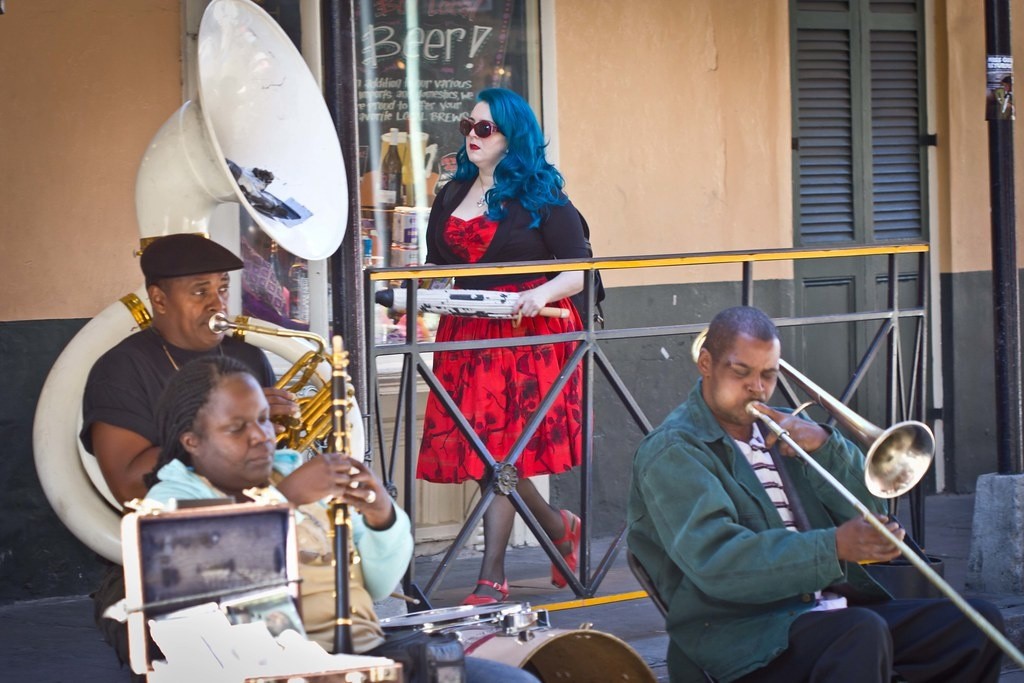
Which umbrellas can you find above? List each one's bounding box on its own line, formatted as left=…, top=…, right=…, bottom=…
left=375, top=288, right=572, bottom=321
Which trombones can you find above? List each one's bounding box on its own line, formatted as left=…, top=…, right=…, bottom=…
left=690, top=326, right=1023, bottom=671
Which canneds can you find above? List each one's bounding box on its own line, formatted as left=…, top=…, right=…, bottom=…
left=390, top=205, right=432, bottom=270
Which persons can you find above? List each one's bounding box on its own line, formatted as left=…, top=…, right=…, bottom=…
left=628, top=307, right=1004, bottom=683
left=378, top=89, right=589, bottom=609
left=75, top=237, right=544, bottom=683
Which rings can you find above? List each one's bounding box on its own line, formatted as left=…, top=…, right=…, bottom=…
left=366, top=490, right=378, bottom=503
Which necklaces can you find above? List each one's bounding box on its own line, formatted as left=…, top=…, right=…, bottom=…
left=155, top=327, right=225, bottom=370
left=477, top=181, right=490, bottom=209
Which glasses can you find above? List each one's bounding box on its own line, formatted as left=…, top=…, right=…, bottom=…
left=459, top=116, right=500, bottom=139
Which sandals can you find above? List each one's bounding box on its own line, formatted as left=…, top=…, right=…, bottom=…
left=464, top=575, right=508, bottom=605
left=550, top=509, right=581, bottom=589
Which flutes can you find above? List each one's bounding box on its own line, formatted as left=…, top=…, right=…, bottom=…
left=331, top=333, right=354, bottom=654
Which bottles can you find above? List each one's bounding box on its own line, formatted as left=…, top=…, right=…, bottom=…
left=362, top=219, right=372, bottom=270
left=287, top=255, right=311, bottom=326
left=382, top=126, right=404, bottom=208
left=269, top=240, right=284, bottom=287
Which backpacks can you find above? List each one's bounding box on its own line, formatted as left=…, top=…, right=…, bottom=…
left=539, top=194, right=606, bottom=313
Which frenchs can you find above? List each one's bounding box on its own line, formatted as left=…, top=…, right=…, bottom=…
left=29, top=0, right=349, bottom=564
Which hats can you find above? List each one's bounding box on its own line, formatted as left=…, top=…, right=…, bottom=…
left=141, top=234, right=243, bottom=279
